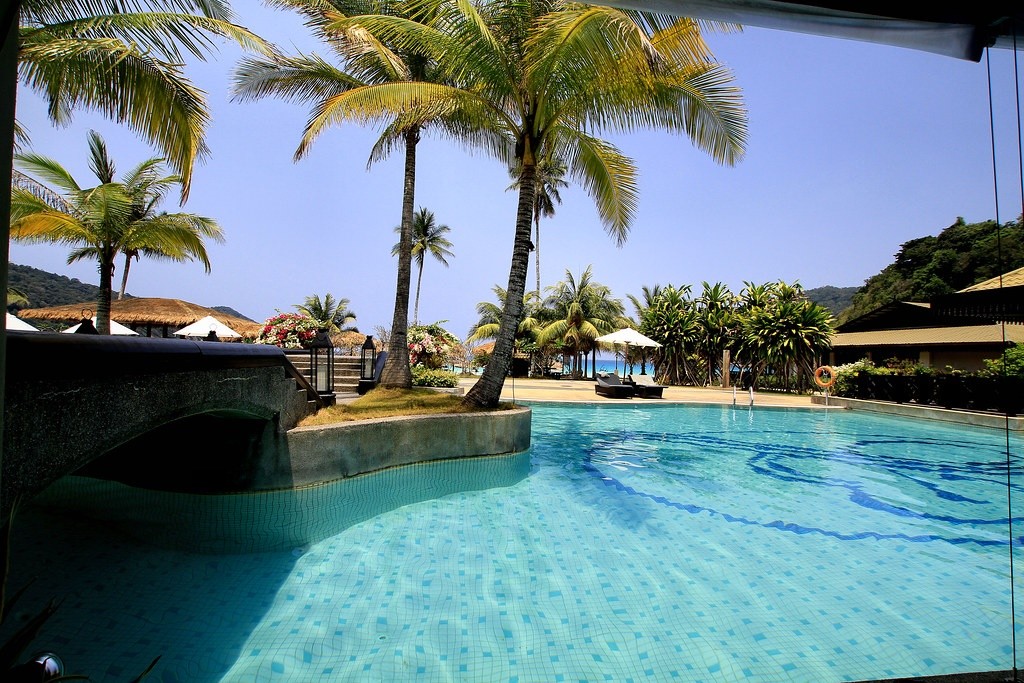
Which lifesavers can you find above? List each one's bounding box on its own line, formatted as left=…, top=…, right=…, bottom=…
left=814, top=366, right=835, bottom=387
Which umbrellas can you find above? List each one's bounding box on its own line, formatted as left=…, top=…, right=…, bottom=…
left=595, top=328, right=663, bottom=381
left=332, top=330, right=376, bottom=355
left=473, top=340, right=531, bottom=359
left=6, top=313, right=39, bottom=331
left=62, top=317, right=139, bottom=335
left=173, top=316, right=243, bottom=338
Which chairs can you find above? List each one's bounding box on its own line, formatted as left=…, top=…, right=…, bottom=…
left=595, top=373, right=634, bottom=399
left=628, top=375, right=669, bottom=399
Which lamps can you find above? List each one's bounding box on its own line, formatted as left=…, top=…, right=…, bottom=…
left=309, top=329, right=334, bottom=394
left=360, top=335, right=376, bottom=380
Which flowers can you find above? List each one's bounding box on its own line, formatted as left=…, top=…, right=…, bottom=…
left=252, top=309, right=317, bottom=350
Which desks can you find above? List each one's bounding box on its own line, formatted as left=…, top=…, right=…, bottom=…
left=622, top=381, right=636, bottom=396
